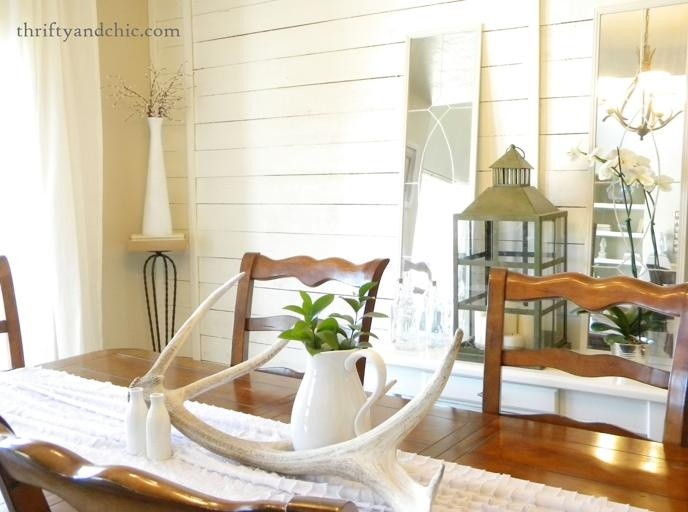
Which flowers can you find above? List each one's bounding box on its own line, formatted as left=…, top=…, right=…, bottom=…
left=593, top=145, right=677, bottom=345
left=568, top=144, right=654, bottom=347
left=101, top=56, right=195, bottom=126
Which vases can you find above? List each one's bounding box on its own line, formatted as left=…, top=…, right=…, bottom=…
left=611, top=337, right=655, bottom=365
left=143, top=113, right=171, bottom=238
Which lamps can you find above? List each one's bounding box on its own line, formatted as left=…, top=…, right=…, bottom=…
left=602, top=9, right=685, bottom=139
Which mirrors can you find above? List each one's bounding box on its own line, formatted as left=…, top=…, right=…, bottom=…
left=576, top=1, right=686, bottom=358
left=396, top=23, right=485, bottom=345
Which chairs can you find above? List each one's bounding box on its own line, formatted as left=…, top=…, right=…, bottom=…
left=475, top=264, right=687, bottom=448
left=231, top=251, right=391, bottom=379
left=0, top=417, right=352, bottom=512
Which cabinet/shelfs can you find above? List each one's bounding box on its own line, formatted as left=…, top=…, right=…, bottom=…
left=565, top=149, right=681, bottom=346
left=453, top=144, right=568, bottom=364
left=396, top=368, right=666, bottom=444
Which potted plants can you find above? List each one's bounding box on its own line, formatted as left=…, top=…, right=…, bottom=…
left=281, top=280, right=393, bottom=451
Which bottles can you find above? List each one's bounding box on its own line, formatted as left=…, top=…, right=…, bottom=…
left=123, top=385, right=172, bottom=460
